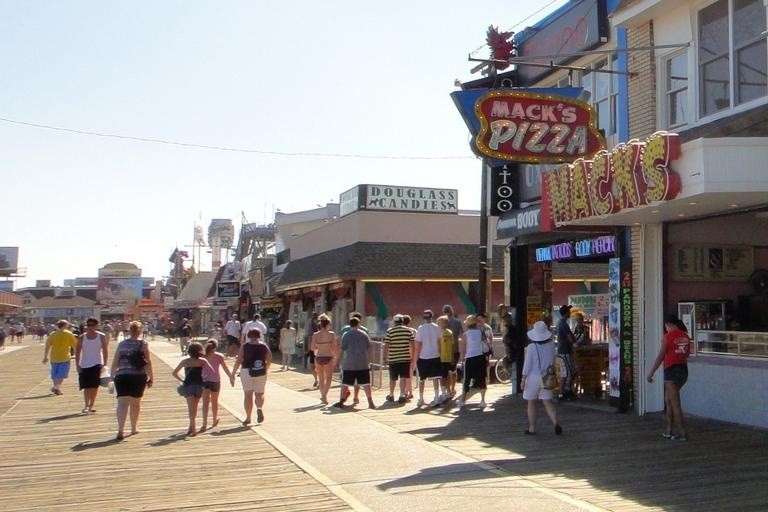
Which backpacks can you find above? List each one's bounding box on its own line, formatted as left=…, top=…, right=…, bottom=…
left=244, top=342, right=265, bottom=377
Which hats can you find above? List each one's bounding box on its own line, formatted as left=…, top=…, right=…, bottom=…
left=560, top=304, right=572, bottom=315
left=527, top=321, right=553, bottom=342
left=393, top=310, right=481, bottom=326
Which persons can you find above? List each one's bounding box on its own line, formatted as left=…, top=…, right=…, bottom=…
left=200, top=339, right=234, bottom=431
left=0, top=321, right=59, bottom=350
left=75, top=317, right=109, bottom=412
left=230, top=328, right=272, bottom=424
left=304, top=312, right=377, bottom=409
left=109, top=320, right=154, bottom=440
left=556, top=304, right=576, bottom=399
left=647, top=313, right=691, bottom=442
left=41, top=320, right=77, bottom=395
left=382, top=303, right=521, bottom=409
left=172, top=342, right=216, bottom=436
left=572, top=312, right=592, bottom=346
left=520, top=320, right=563, bottom=436
left=65, top=309, right=296, bottom=370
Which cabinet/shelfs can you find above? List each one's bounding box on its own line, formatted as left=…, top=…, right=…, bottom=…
left=678, top=300, right=728, bottom=357
left=697, top=330, right=768, bottom=358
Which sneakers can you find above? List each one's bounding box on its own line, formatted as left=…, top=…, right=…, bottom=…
left=257, top=408, right=264, bottom=424
left=524, top=429, right=535, bottom=434
left=661, top=430, right=673, bottom=439
left=554, top=424, right=563, bottom=434
left=333, top=390, right=487, bottom=411
left=671, top=433, right=688, bottom=441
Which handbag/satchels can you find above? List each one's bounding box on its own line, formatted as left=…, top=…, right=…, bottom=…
left=128, top=351, right=147, bottom=369
left=177, top=383, right=185, bottom=396
left=541, top=373, right=560, bottom=390
